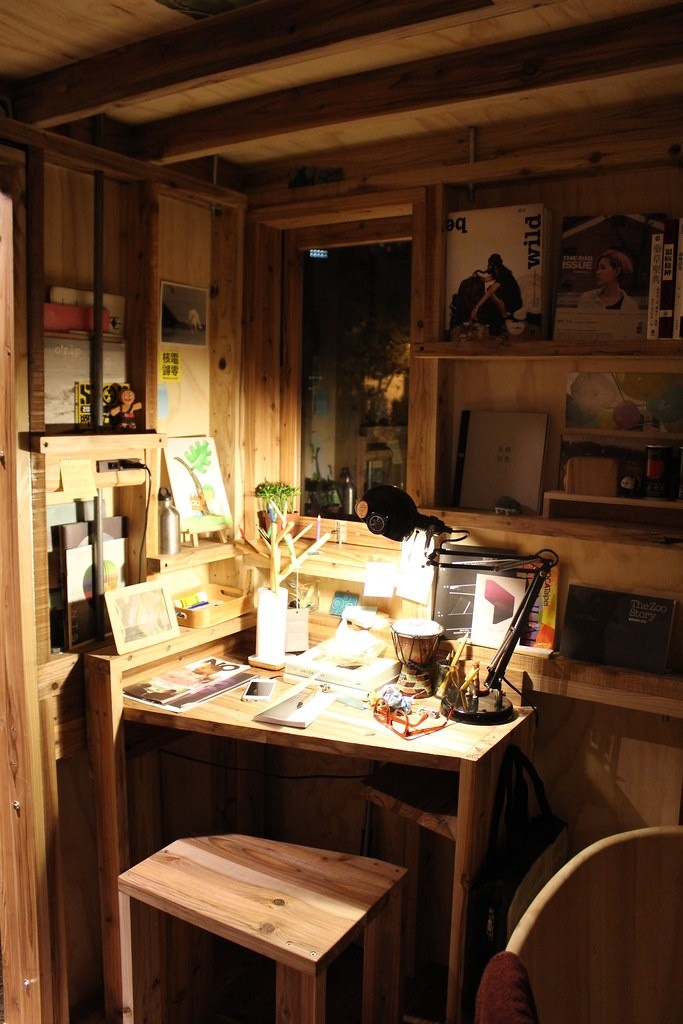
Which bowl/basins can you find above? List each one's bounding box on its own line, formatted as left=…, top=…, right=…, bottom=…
left=506, top=322, right=525, bottom=335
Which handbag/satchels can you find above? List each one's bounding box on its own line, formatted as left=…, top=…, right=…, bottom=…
left=468, top=745, right=570, bottom=957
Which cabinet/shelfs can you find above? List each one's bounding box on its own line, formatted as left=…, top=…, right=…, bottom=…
left=0, top=114, right=250, bottom=664
left=410, top=156, right=683, bottom=719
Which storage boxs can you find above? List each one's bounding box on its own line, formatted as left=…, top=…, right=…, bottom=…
left=173, top=583, right=252, bottom=629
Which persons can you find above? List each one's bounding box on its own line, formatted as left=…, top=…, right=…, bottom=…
left=471, top=254, right=523, bottom=321
left=578, top=250, right=639, bottom=311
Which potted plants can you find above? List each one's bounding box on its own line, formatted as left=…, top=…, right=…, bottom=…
left=304, top=475, right=342, bottom=519
left=254, top=482, right=302, bottom=541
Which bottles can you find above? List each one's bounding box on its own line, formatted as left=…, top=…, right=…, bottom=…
left=158, top=488, right=182, bottom=555
left=339, top=467, right=355, bottom=516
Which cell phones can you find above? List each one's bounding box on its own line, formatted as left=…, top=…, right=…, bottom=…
left=242, top=679, right=275, bottom=702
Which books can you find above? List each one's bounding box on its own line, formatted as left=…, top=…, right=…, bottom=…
left=254, top=672, right=338, bottom=728
left=451, top=410, right=550, bottom=515
left=553, top=213, right=683, bottom=340
left=433, top=544, right=559, bottom=659
left=559, top=584, right=677, bottom=676
left=60, top=515, right=129, bottom=653
left=443, top=204, right=554, bottom=342
left=122, top=656, right=258, bottom=713
left=283, top=646, right=401, bottom=710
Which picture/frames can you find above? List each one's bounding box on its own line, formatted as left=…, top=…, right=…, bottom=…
left=104, top=579, right=181, bottom=655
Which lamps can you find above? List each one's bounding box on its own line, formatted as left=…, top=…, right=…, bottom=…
left=353, top=483, right=561, bottom=726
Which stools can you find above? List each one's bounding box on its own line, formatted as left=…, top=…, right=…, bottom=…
left=117, top=833, right=411, bottom=1024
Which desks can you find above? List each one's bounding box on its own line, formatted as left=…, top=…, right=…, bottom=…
left=87, top=609, right=537, bottom=1024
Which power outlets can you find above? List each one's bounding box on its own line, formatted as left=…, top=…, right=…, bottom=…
left=118, top=457, right=139, bottom=471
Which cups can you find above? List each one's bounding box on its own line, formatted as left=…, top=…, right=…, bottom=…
left=642, top=443, right=675, bottom=501
